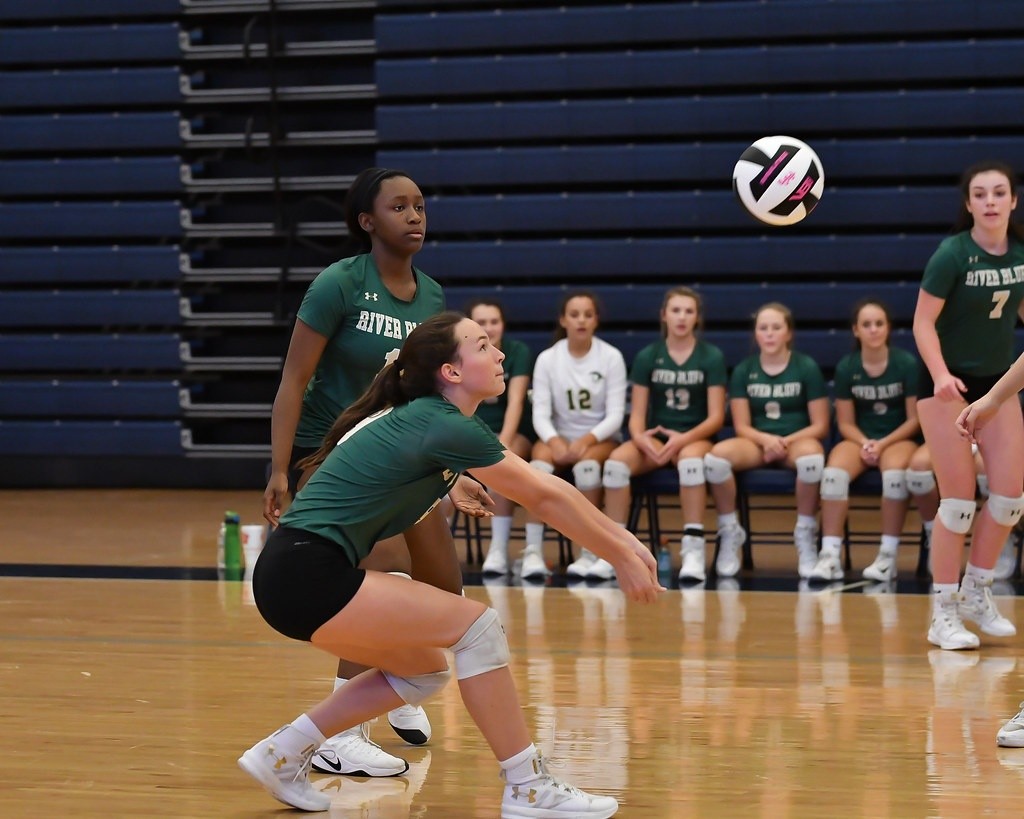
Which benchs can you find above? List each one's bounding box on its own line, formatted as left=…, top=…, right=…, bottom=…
left=0, top=0, right=1024, bottom=456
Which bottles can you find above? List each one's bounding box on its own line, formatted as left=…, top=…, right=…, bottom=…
left=215, top=521, right=226, bottom=568
left=656, top=537, right=673, bottom=590
left=224, top=510, right=241, bottom=569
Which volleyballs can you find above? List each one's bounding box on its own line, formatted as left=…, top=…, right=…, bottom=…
left=731, top=134, right=826, bottom=227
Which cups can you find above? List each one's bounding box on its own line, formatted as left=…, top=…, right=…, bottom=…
left=241, top=525, right=264, bottom=568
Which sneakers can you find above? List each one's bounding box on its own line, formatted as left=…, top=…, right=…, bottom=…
left=237, top=724, right=330, bottom=811
left=679, top=535, right=705, bottom=581
left=311, top=778, right=408, bottom=811
left=928, top=650, right=979, bottom=684
left=811, top=545, right=845, bottom=582
left=715, top=522, right=746, bottom=577
left=501, top=749, right=619, bottom=819
left=997, top=701, right=1024, bottom=747
left=310, top=720, right=408, bottom=777
left=482, top=543, right=507, bottom=574
left=585, top=557, right=616, bottom=579
left=717, top=578, right=746, bottom=621
left=520, top=545, right=545, bottom=578
left=925, top=537, right=934, bottom=574
left=794, top=525, right=820, bottom=578
left=483, top=576, right=508, bottom=595
left=800, top=581, right=841, bottom=602
left=863, top=582, right=896, bottom=601
left=927, top=594, right=980, bottom=650
left=566, top=551, right=597, bottom=578
left=523, top=578, right=545, bottom=600
left=388, top=703, right=431, bottom=744
left=567, top=579, right=624, bottom=601
left=979, top=657, right=1016, bottom=679
left=993, top=534, right=1018, bottom=580
left=679, top=580, right=706, bottom=608
left=863, top=548, right=897, bottom=581
left=399, top=750, right=431, bottom=794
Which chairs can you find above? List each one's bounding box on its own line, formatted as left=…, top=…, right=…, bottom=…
left=449, top=418, right=1024, bottom=587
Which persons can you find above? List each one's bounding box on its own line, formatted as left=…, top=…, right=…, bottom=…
left=236, top=309, right=668, bottom=819
left=589, top=286, right=730, bottom=580
left=262, top=167, right=494, bottom=778
left=704, top=303, right=830, bottom=578
left=811, top=300, right=926, bottom=581
left=518, top=294, right=625, bottom=579
left=466, top=297, right=536, bottom=574
left=907, top=439, right=1017, bottom=579
left=909, top=168, right=1024, bottom=649
